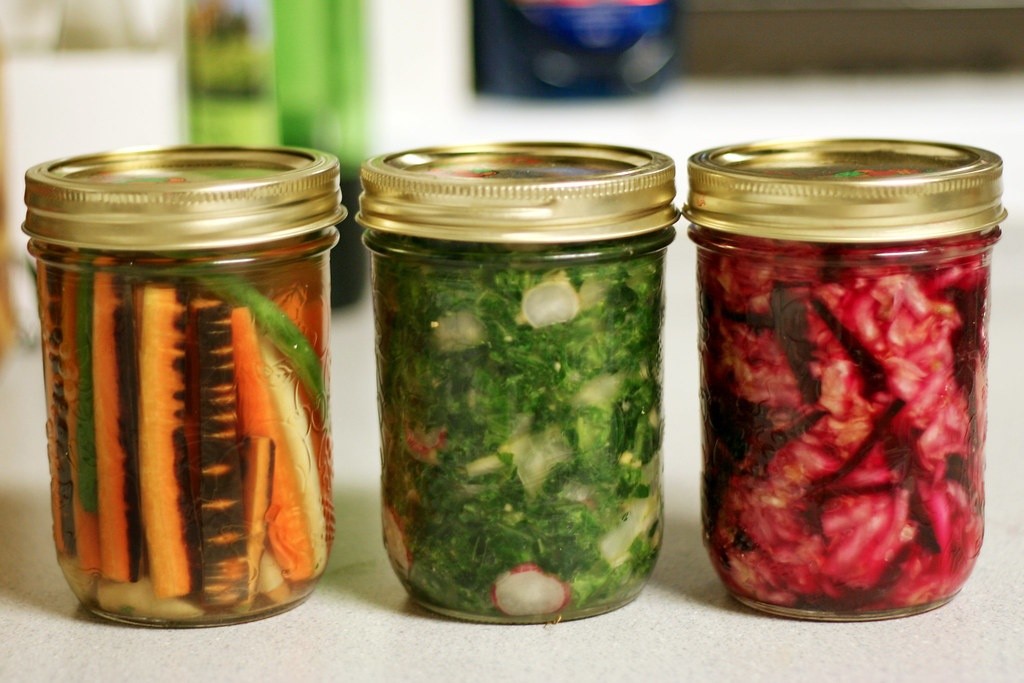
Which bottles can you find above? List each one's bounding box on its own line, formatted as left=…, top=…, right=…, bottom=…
left=682, top=137, right=1009, bottom=622
left=183, top=0, right=370, bottom=308
left=19, top=142, right=348, bottom=629
left=352, top=140, right=682, bottom=624
